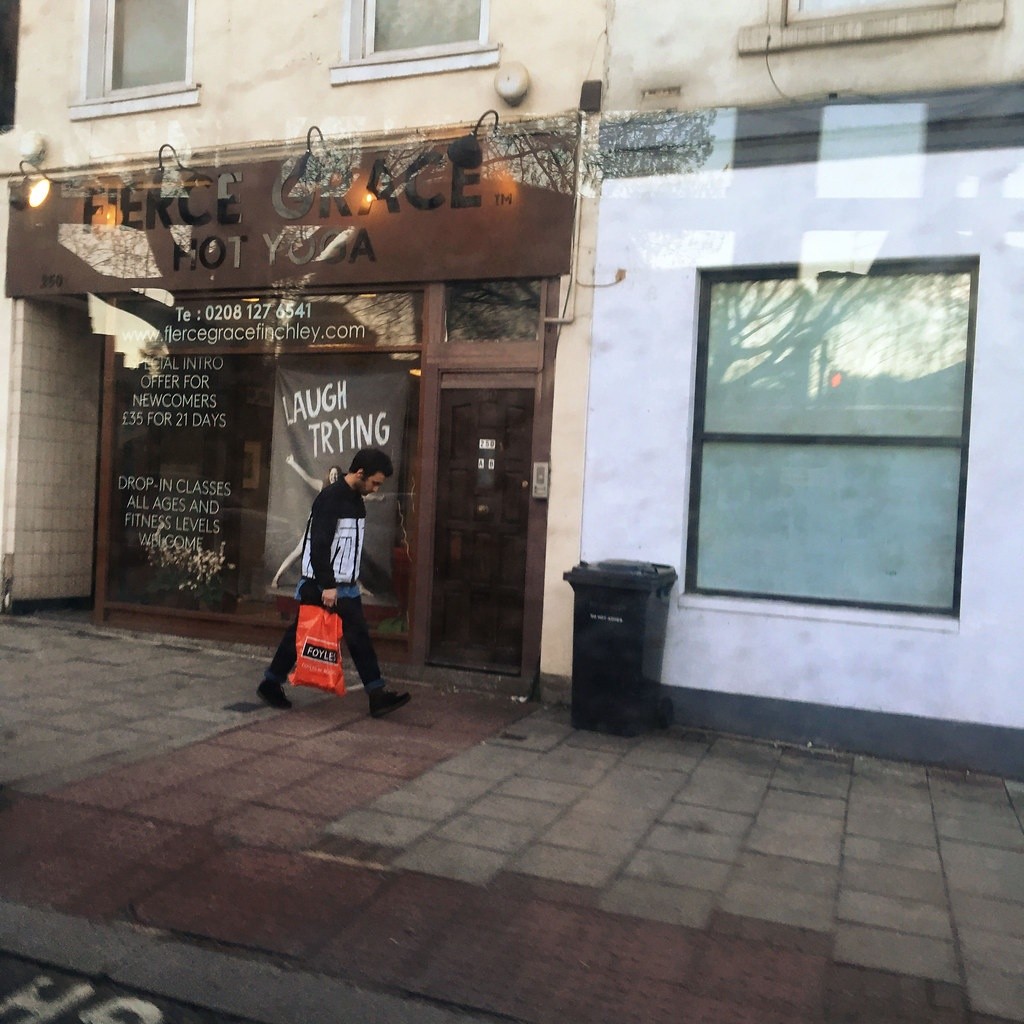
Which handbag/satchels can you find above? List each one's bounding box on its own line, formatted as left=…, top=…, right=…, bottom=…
left=287, top=604, right=347, bottom=696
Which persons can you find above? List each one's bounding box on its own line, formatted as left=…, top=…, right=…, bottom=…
left=270, top=454, right=385, bottom=597
left=256, top=448, right=411, bottom=719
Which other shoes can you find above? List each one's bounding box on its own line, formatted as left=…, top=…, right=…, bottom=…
left=256, top=680, right=292, bottom=709
left=369, top=688, right=411, bottom=718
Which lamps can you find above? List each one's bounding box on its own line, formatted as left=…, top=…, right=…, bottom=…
left=447, top=110, right=499, bottom=168
left=296, top=125, right=334, bottom=182
left=19, top=160, right=72, bottom=209
left=149, top=143, right=195, bottom=200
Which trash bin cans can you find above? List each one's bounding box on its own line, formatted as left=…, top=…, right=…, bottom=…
left=563, top=558, right=680, bottom=735
